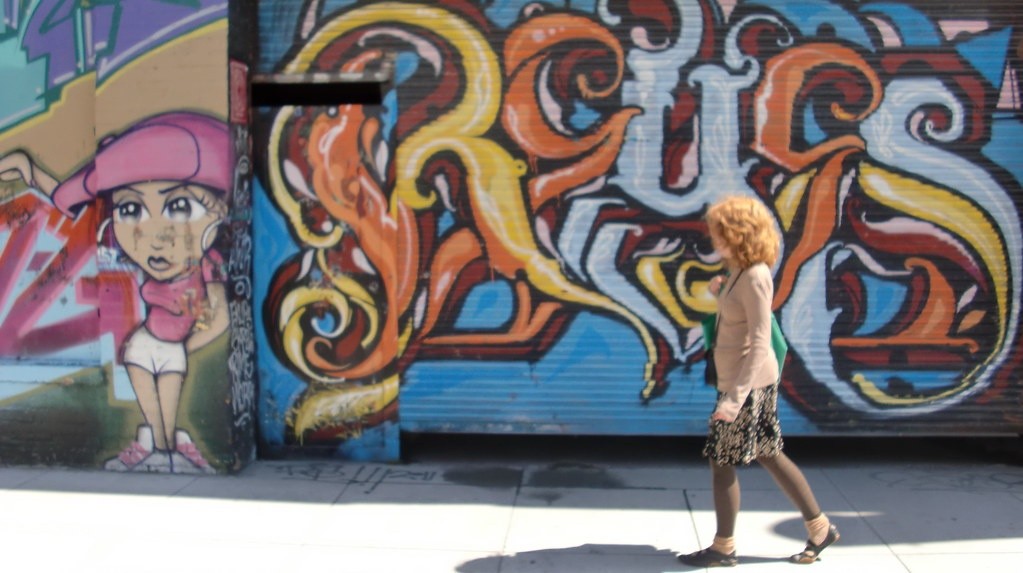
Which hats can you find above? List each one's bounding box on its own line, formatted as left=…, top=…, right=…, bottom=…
left=51, top=111, right=241, bottom=219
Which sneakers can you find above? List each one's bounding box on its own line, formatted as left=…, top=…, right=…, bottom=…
left=170, top=431, right=215, bottom=474
left=678, top=547, right=738, bottom=567
left=102, top=426, right=170, bottom=473
left=790, top=526, right=840, bottom=564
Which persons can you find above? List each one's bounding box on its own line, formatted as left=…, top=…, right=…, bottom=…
left=678, top=194, right=841, bottom=568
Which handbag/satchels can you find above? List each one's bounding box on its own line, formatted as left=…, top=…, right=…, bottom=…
left=700, top=313, right=789, bottom=387
